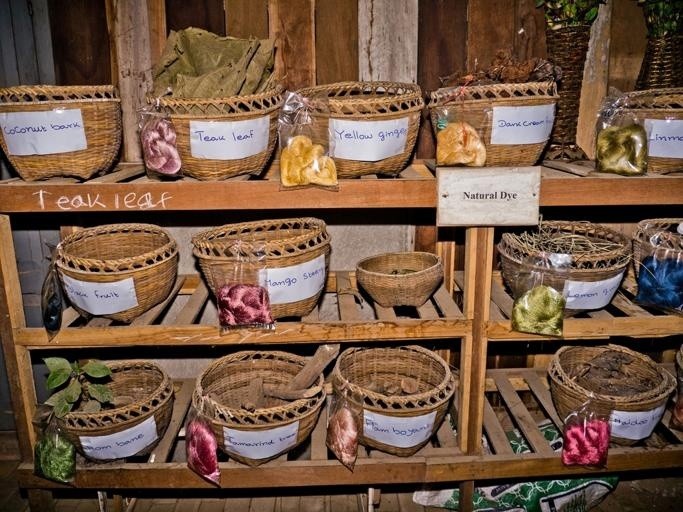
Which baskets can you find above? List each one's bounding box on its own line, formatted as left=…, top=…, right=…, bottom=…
left=53, top=223, right=180, bottom=325
left=497, top=219, right=631, bottom=319
left=545, top=342, right=677, bottom=447
left=190, top=215, right=331, bottom=321
left=50, top=359, right=176, bottom=464
left=143, top=84, right=285, bottom=183
left=428, top=78, right=560, bottom=169
left=190, top=350, right=328, bottom=468
left=289, top=81, right=425, bottom=176
left=331, top=342, right=456, bottom=458
left=354, top=250, right=445, bottom=308
left=0, top=83, right=122, bottom=183
left=631, top=216, right=682, bottom=313
left=612, top=88, right=683, bottom=177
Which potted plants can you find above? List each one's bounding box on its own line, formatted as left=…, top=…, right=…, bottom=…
left=533, top=0, right=682, bottom=162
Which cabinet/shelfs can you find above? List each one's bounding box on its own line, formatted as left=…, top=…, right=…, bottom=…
left=1, top=155, right=682, bottom=511
